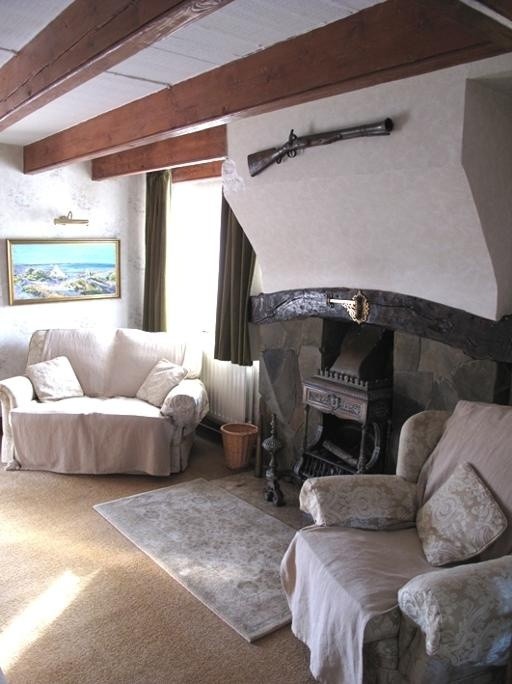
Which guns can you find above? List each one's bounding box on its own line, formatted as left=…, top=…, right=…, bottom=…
left=247, top=117, right=394, bottom=177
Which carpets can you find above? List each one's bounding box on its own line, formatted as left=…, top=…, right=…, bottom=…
left=94, top=474, right=300, bottom=643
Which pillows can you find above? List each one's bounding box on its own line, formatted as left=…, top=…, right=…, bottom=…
left=26, top=355, right=84, bottom=403
left=415, top=460, right=508, bottom=567
left=135, top=355, right=189, bottom=408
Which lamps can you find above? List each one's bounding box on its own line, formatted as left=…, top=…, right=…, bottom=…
left=54, top=212, right=89, bottom=226
left=199, top=351, right=255, bottom=428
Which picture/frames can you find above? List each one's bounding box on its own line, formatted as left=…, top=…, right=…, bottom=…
left=5, top=237, right=121, bottom=305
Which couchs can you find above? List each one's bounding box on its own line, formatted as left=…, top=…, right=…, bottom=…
left=0, top=327, right=209, bottom=477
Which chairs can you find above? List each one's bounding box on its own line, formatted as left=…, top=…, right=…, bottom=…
left=278, top=398, right=511, bottom=684
